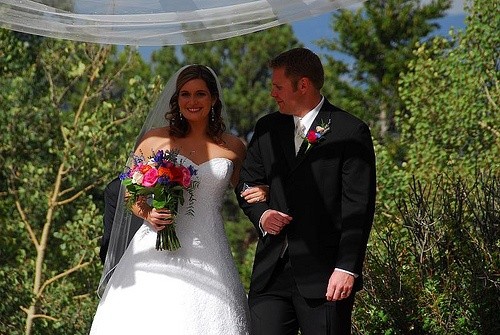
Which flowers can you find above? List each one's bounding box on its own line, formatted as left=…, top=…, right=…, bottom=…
left=299, top=118, right=332, bottom=155
left=120, top=144, right=202, bottom=252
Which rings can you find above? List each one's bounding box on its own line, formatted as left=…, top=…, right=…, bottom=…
left=256, top=198, right=259, bottom=202
left=341, top=291, right=347, bottom=295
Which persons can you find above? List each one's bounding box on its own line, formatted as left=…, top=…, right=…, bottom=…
left=234, top=48, right=378, bottom=334
left=89, top=63, right=270, bottom=334
